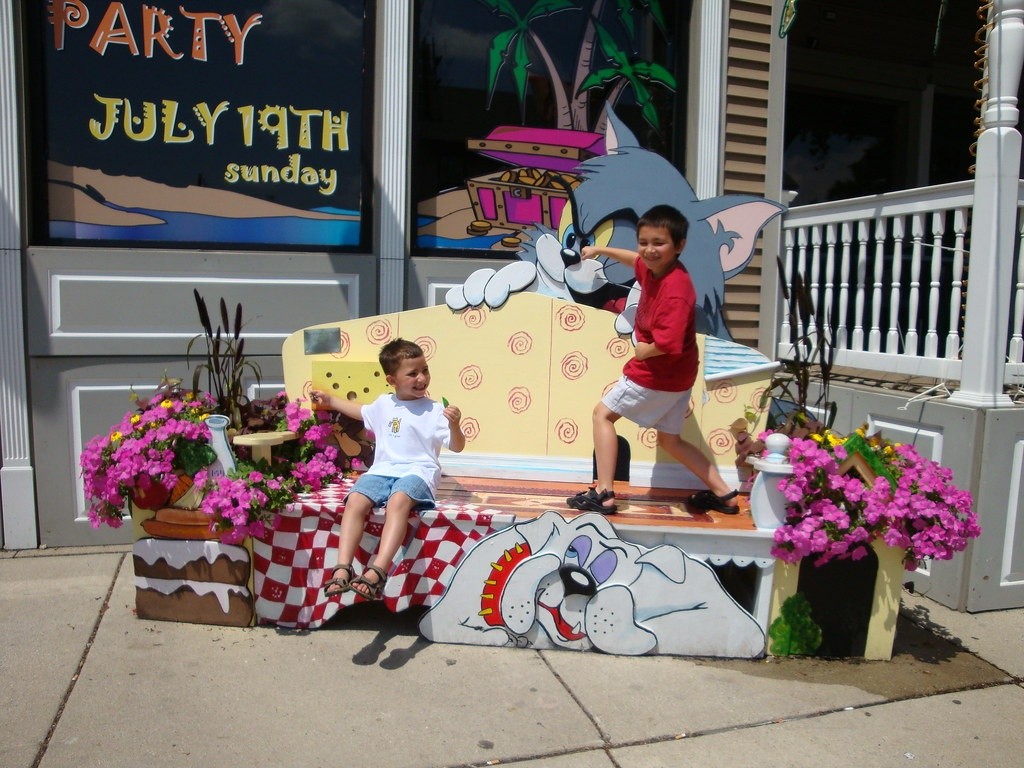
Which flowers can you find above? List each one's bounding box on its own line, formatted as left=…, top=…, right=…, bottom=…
left=79, top=285, right=377, bottom=547
left=755, top=254, right=984, bottom=572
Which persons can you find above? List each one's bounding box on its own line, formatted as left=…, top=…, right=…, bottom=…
left=309, top=339, right=465, bottom=600
left=566, top=205, right=740, bottom=515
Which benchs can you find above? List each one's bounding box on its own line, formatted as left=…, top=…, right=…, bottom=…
left=232, top=293, right=797, bottom=661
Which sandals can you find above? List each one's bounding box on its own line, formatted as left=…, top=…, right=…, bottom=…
left=689, top=488, right=740, bottom=514
left=349, top=566, right=387, bottom=600
left=566, top=486, right=617, bottom=515
left=324, top=565, right=355, bottom=596
